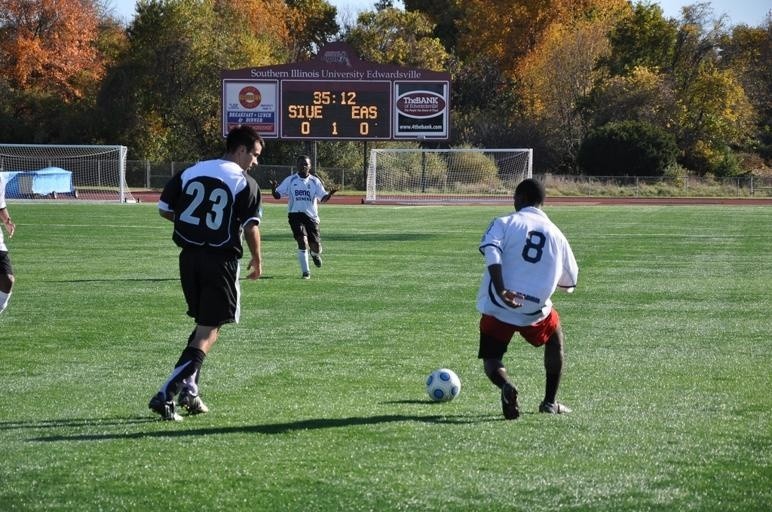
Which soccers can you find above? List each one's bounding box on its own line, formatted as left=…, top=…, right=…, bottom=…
left=427, top=367, right=461, bottom=405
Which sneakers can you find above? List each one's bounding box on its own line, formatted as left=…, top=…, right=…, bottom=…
left=149, top=392, right=186, bottom=423
left=302, top=272, right=311, bottom=280
left=538, top=399, right=573, bottom=415
left=180, top=386, right=209, bottom=414
left=502, top=382, right=520, bottom=420
left=309, top=249, right=323, bottom=268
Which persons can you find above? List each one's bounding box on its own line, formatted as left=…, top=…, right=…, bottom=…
left=478, top=178, right=579, bottom=419
left=269, top=155, right=339, bottom=280
left=0, top=175, right=16, bottom=314
left=149, top=124, right=263, bottom=422
